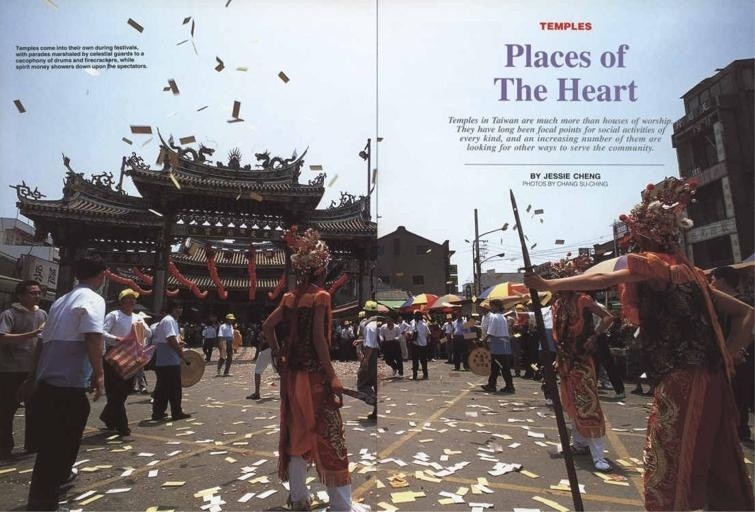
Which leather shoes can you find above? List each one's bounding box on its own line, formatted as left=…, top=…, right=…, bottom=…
left=120, top=428, right=131, bottom=434
left=100, top=414, right=114, bottom=430
left=152, top=413, right=168, bottom=420
left=172, top=413, right=190, bottom=419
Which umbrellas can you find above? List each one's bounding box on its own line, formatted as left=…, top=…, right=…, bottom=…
left=396, top=278, right=550, bottom=314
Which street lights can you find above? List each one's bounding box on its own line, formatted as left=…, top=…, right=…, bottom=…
left=117, top=155, right=132, bottom=191
left=359, top=136, right=377, bottom=300
left=471, top=222, right=510, bottom=298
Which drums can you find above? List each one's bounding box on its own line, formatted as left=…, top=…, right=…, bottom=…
left=464, top=340, right=491, bottom=375
left=180, top=348, right=205, bottom=388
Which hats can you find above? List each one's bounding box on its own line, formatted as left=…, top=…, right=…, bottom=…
left=118, top=288, right=140, bottom=302
left=478, top=303, right=491, bottom=311
left=225, top=313, right=237, bottom=320
left=618, top=172, right=700, bottom=249
left=358, top=311, right=366, bottom=318
left=279, top=225, right=331, bottom=276
left=362, top=300, right=378, bottom=312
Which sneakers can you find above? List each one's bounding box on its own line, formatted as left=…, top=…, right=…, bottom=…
left=567, top=446, right=584, bottom=454
left=631, top=388, right=642, bottom=394
left=500, top=386, right=515, bottom=393
left=616, top=387, right=625, bottom=399
left=644, top=392, right=652, bottom=396
left=246, top=393, right=260, bottom=399
left=481, top=385, right=496, bottom=391
left=593, top=457, right=612, bottom=472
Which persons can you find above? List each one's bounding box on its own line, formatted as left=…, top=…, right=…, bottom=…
left=352, top=300, right=378, bottom=428
left=707, top=264, right=755, bottom=441
left=150, top=297, right=192, bottom=421
left=261, top=246, right=372, bottom=512
left=243, top=302, right=287, bottom=400
left=87, top=310, right=244, bottom=394
left=522, top=216, right=755, bottom=511
left=0, top=278, right=49, bottom=458
left=544, top=271, right=616, bottom=471
left=335, top=300, right=658, bottom=395
left=98, top=287, right=153, bottom=437
left=14, top=252, right=109, bottom=512
